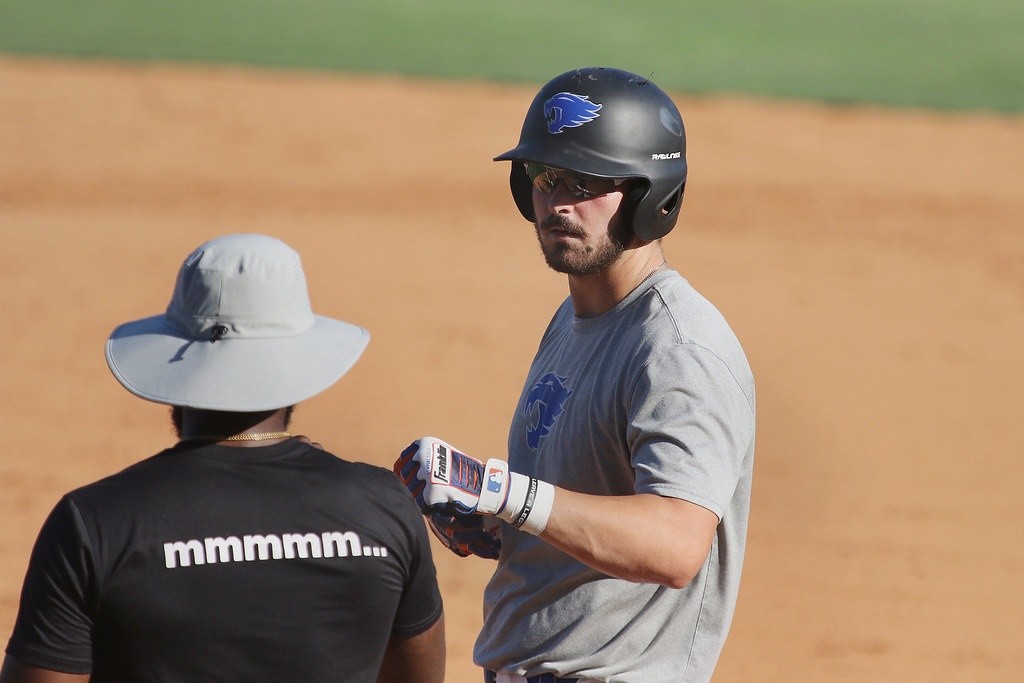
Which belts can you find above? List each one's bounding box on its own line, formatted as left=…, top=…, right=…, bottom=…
left=484, top=668, right=579, bottom=683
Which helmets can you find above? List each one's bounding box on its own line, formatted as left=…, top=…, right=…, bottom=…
left=492, top=67, right=689, bottom=241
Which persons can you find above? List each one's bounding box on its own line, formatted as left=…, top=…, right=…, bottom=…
left=0, top=233, right=446, bottom=683
left=392, top=67, right=757, bottom=683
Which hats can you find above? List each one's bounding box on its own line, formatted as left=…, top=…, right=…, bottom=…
left=105, top=234, right=371, bottom=411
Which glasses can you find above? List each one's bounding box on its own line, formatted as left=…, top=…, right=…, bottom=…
left=523, top=161, right=634, bottom=198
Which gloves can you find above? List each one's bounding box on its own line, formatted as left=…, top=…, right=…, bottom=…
left=393, top=436, right=555, bottom=558
